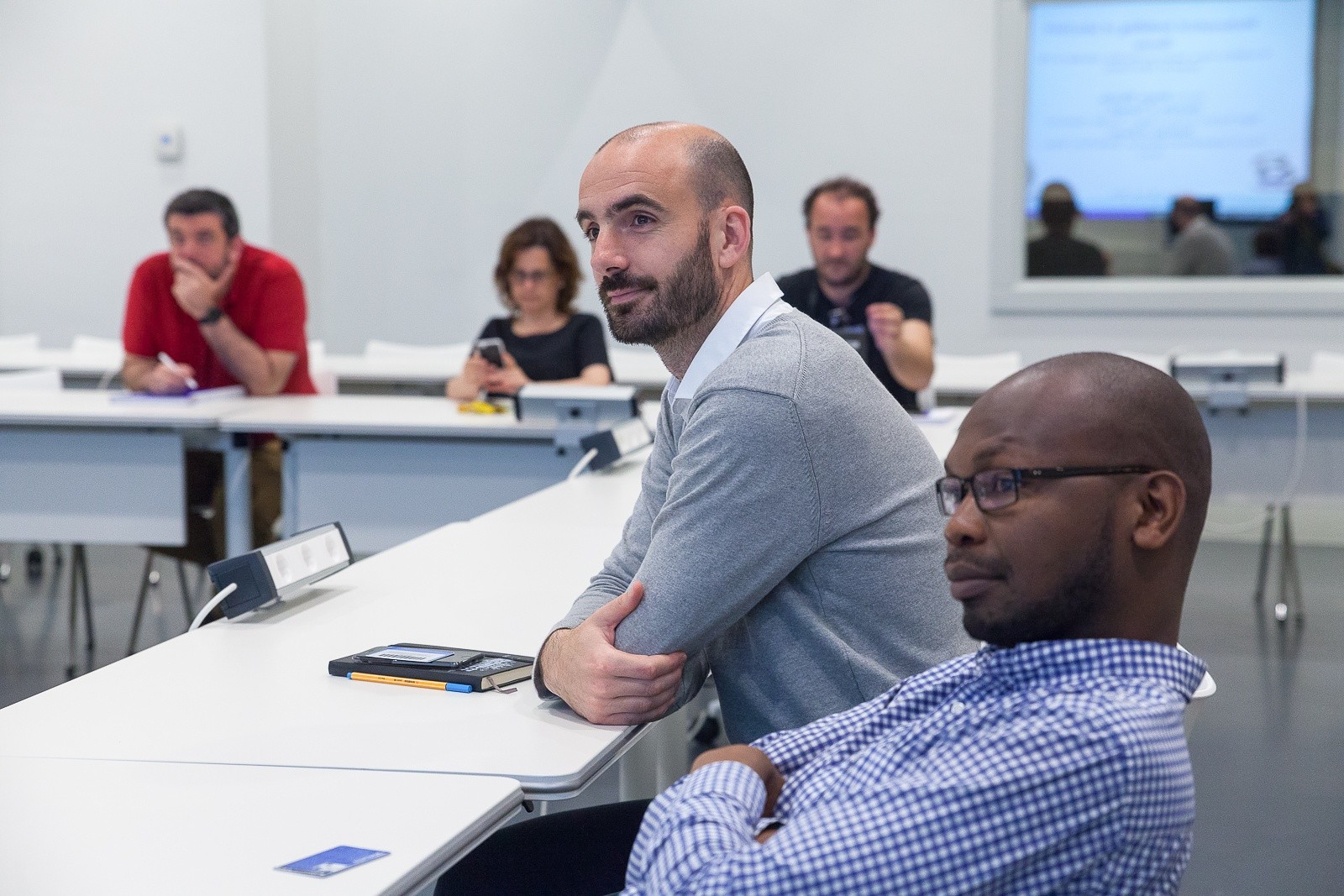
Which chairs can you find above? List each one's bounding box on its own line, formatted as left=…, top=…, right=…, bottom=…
left=935, top=355, right=1021, bottom=394
left=1169, top=351, right=1343, bottom=637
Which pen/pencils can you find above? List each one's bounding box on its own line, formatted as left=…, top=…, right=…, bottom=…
left=158, top=350, right=202, bottom=391
left=347, top=672, right=472, bottom=693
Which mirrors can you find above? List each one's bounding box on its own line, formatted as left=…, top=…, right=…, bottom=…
left=993, top=0, right=1344, bottom=323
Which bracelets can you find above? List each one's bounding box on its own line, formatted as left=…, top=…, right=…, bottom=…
left=196, top=308, right=222, bottom=325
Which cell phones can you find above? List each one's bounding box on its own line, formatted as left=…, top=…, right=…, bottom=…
left=476, top=339, right=506, bottom=369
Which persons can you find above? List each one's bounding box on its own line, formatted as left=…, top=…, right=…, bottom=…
left=613, top=354, right=1210, bottom=896
left=777, top=179, right=933, bottom=412
left=444, top=217, right=612, bottom=402
left=1256, top=182, right=1344, bottom=275
left=1160, top=199, right=1250, bottom=278
left=122, top=190, right=318, bottom=565
left=1027, top=183, right=1105, bottom=276
left=430, top=121, right=983, bottom=896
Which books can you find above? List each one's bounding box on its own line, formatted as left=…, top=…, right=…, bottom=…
left=327, top=643, right=535, bottom=692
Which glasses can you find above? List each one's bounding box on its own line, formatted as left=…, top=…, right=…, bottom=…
left=934, top=465, right=1159, bottom=518
left=509, top=269, right=555, bottom=284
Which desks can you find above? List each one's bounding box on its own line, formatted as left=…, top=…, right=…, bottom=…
left=1, top=758, right=524, bottom=894
left=220, top=393, right=661, bottom=557
left=1, top=391, right=243, bottom=427
left=0, top=522, right=660, bottom=817
left=473, top=427, right=972, bottom=522
left=637, top=399, right=972, bottom=439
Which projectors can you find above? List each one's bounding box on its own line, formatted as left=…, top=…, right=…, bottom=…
left=1169, top=351, right=1282, bottom=383
